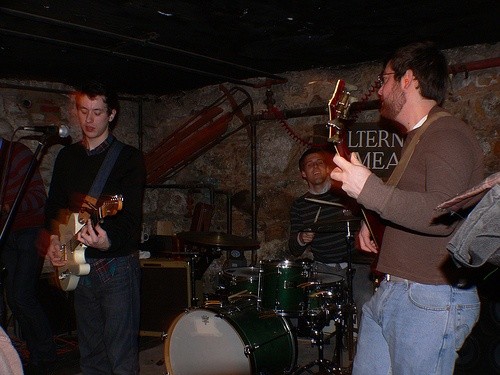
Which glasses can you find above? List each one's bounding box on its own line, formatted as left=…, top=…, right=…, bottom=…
left=377, top=72, right=395, bottom=85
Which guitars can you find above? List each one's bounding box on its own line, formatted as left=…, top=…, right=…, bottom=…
left=326, top=80, right=380, bottom=249
left=54, top=193, right=123, bottom=291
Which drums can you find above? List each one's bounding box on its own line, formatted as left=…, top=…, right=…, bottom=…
left=259, top=258, right=309, bottom=318
left=160, top=297, right=299, bottom=375
left=223, top=267, right=260, bottom=294
left=308, top=273, right=349, bottom=309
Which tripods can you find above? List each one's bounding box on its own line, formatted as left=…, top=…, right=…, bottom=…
left=293, top=322, right=344, bottom=375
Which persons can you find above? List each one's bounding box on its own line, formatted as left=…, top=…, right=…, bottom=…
left=288, top=148, right=372, bottom=332
left=0, top=136, right=57, bottom=375
left=329, top=41, right=483, bottom=375
left=46, top=79, right=146, bottom=375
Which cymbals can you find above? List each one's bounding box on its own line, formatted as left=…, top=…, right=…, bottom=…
left=174, top=230, right=259, bottom=248
left=302, top=211, right=363, bottom=226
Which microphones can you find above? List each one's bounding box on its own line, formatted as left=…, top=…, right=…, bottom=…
left=18, top=125, right=70, bottom=138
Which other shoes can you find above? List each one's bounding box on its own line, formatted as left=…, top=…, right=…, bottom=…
left=344, top=313, right=358, bottom=344
left=311, top=320, right=337, bottom=343
